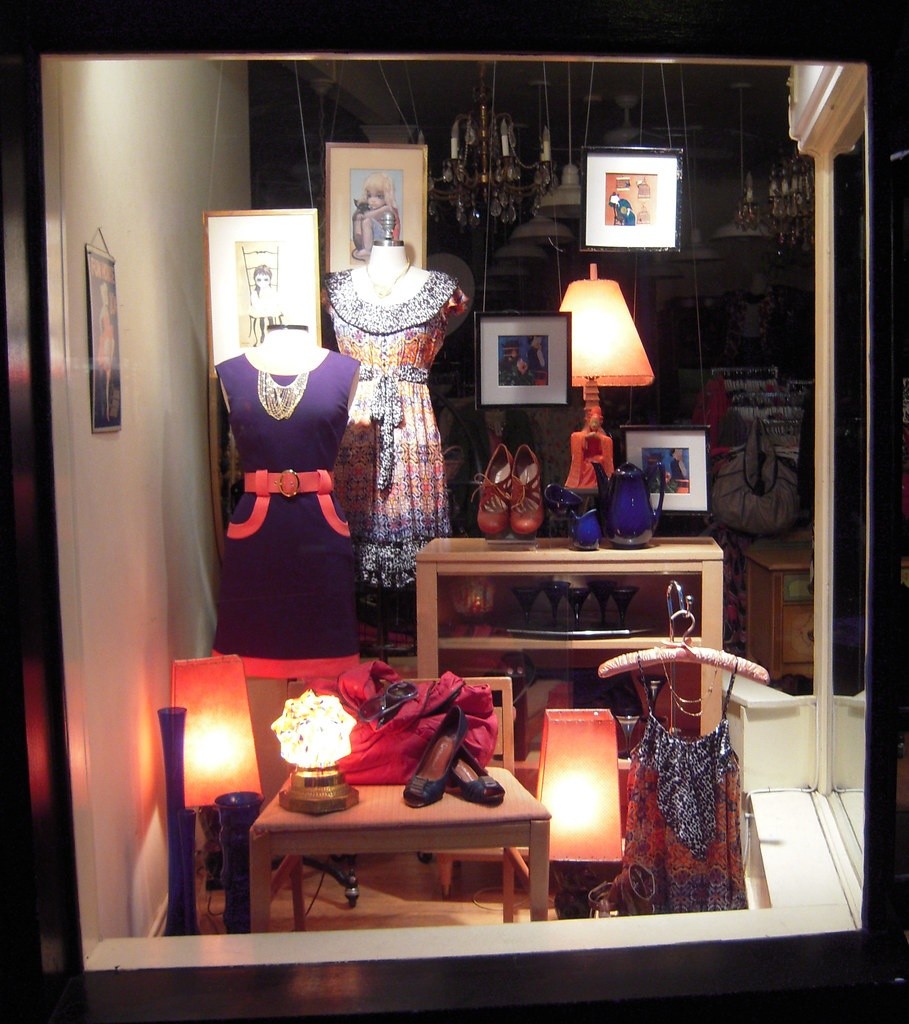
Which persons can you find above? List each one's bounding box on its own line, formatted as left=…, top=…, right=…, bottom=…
left=564, top=407, right=614, bottom=489
left=211, top=325, right=359, bottom=679
left=320, top=246, right=469, bottom=586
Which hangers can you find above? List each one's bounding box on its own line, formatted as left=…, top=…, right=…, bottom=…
left=597, top=612, right=772, bottom=687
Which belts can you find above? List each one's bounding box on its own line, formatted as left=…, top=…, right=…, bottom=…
left=225, top=469, right=350, bottom=541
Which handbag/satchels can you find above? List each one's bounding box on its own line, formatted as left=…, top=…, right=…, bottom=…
left=334, top=657, right=499, bottom=784
left=712, top=417, right=801, bottom=537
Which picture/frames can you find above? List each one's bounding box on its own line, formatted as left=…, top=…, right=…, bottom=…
left=473, top=311, right=573, bottom=406
left=204, top=205, right=322, bottom=376
left=325, top=142, right=430, bottom=274
left=580, top=145, right=684, bottom=252
left=622, top=424, right=713, bottom=515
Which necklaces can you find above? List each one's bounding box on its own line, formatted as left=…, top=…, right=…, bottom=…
left=659, top=648, right=719, bottom=716
left=256, top=349, right=312, bottom=425
left=365, top=256, right=410, bottom=299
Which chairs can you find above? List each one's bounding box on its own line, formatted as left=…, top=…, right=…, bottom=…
left=397, top=677, right=531, bottom=893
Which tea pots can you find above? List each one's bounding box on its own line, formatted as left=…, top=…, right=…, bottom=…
left=591, top=461, right=666, bottom=545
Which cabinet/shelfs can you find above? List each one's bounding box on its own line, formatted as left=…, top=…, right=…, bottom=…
left=745, top=545, right=815, bottom=680
left=414, top=537, right=725, bottom=735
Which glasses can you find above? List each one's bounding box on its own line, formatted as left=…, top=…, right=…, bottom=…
left=358, top=679, right=419, bottom=730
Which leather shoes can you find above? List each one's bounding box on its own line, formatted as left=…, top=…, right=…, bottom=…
left=471, top=443, right=545, bottom=537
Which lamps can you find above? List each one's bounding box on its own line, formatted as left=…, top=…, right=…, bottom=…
left=557, top=263, right=654, bottom=494
left=492, top=243, right=553, bottom=268
left=512, top=213, right=574, bottom=248
left=426, top=60, right=559, bottom=223
left=710, top=76, right=769, bottom=242
left=536, top=62, right=578, bottom=218
left=271, top=690, right=359, bottom=813
left=488, top=265, right=528, bottom=278
left=535, top=710, right=624, bottom=919
left=676, top=121, right=723, bottom=264
left=170, top=656, right=262, bottom=890
left=738, top=147, right=815, bottom=251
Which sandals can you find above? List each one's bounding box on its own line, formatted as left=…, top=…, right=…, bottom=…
left=404, top=703, right=506, bottom=808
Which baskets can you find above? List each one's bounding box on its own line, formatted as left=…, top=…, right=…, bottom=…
left=444, top=446, right=464, bottom=481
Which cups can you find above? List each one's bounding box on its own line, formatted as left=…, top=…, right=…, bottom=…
left=570, top=509, right=602, bottom=550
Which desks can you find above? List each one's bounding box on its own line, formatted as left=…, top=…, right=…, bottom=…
left=247, top=766, right=551, bottom=935
